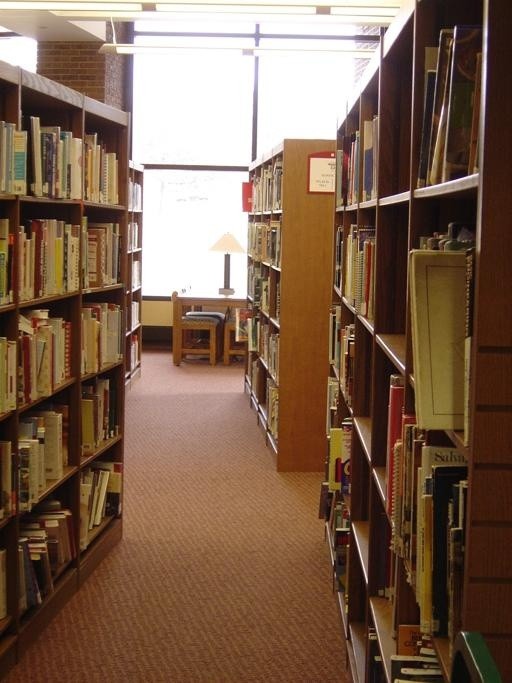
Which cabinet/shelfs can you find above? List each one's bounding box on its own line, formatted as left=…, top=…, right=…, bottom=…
left=0, top=64, right=132, bottom=680
left=0, top=61, right=143, bottom=679
left=129, top=158, right=143, bottom=390
left=246, top=139, right=337, bottom=474
left=320, top=2, right=510, bottom=683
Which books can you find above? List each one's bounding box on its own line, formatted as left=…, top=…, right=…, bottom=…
left=234, top=162, right=282, bottom=440
left=317, top=24, right=483, bottom=683
left=0, top=108, right=141, bottom=631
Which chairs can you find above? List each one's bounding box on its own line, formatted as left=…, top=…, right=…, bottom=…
left=168, top=283, right=251, bottom=361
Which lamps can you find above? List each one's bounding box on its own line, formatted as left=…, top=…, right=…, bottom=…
left=211, top=228, right=241, bottom=294
